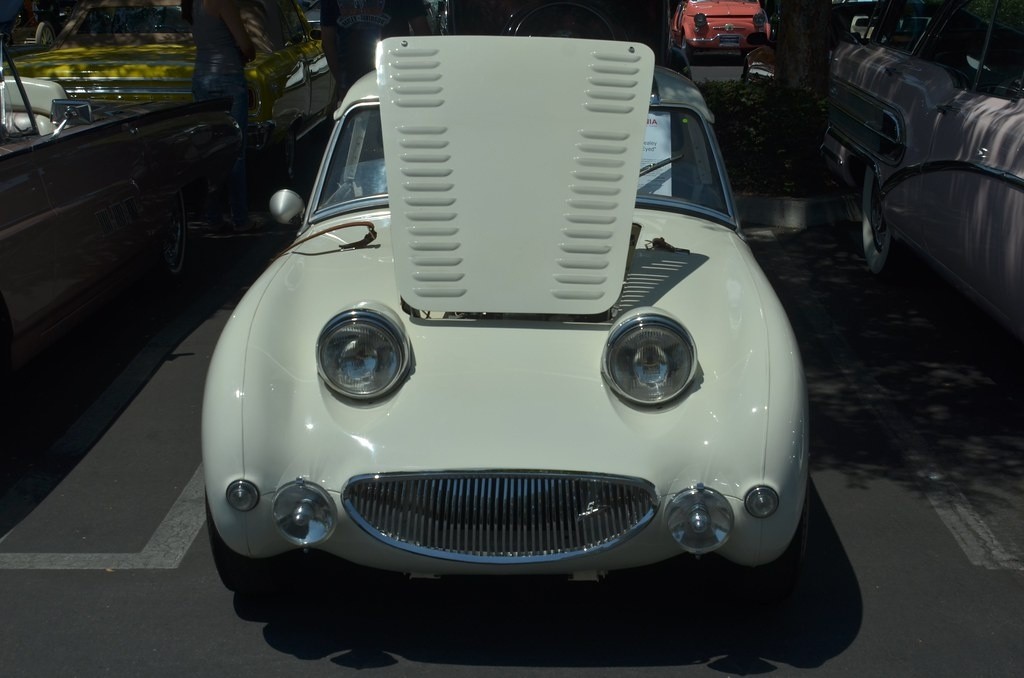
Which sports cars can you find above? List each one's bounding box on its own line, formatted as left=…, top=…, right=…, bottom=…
left=200, top=33, right=814, bottom=616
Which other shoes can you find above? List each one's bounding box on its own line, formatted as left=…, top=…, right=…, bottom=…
left=231, top=214, right=266, bottom=233
left=201, top=221, right=233, bottom=238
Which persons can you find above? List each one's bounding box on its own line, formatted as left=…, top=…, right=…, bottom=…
left=320, top=0, right=432, bottom=108
left=191, top=0, right=256, bottom=159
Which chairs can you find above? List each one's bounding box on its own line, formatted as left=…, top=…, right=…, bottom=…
left=4, top=75, right=70, bottom=136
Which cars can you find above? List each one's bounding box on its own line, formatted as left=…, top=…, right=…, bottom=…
left=0, top=40, right=245, bottom=368
left=1, top=0, right=349, bottom=159
left=664, top=0, right=778, bottom=66
left=811, top=0, right=1023, bottom=351
